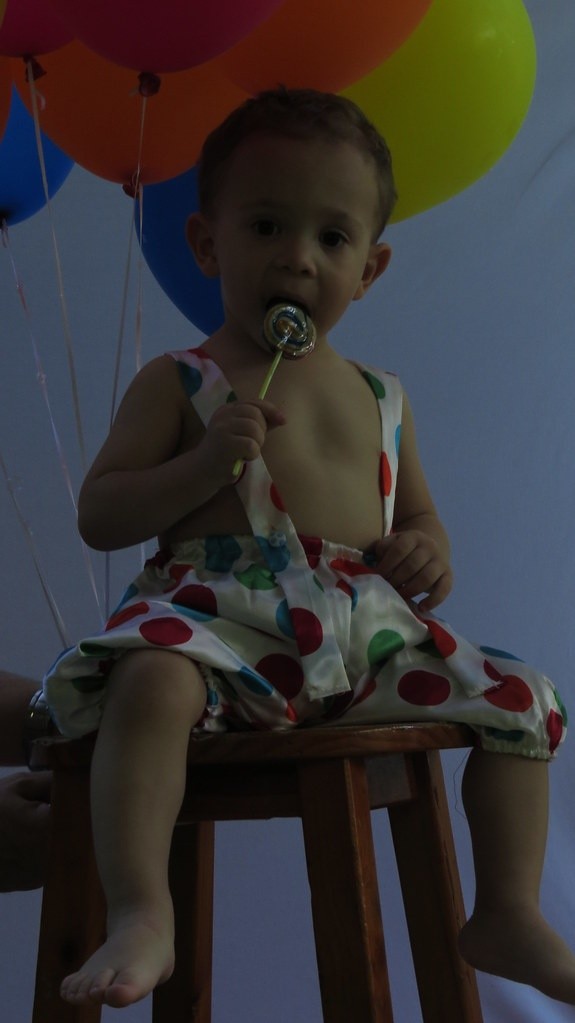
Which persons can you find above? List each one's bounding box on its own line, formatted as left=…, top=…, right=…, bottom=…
left=59, top=88, right=575, bottom=1011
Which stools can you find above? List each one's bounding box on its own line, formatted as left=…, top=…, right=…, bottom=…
left=33, top=721, right=482, bottom=1022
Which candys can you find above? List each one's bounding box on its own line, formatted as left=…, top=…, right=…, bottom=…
left=225, top=305, right=317, bottom=474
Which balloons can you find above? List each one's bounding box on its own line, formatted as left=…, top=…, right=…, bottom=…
left=0, top=1, right=539, bottom=343
